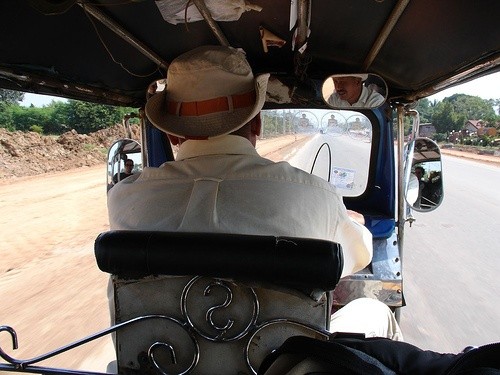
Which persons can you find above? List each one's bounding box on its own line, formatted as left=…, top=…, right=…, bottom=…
left=326, top=74, right=385, bottom=107
left=107, top=44, right=405, bottom=343
left=414, top=167, right=432, bottom=204
left=111, top=159, right=134, bottom=185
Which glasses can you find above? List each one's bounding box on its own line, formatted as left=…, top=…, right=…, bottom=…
left=126, top=164, right=134, bottom=167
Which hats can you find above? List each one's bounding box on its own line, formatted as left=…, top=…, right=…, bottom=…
left=144, top=44, right=270, bottom=140
left=331, top=74, right=368, bottom=81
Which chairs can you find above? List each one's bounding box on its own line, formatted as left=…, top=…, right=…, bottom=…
left=94, top=230, right=344, bottom=375
